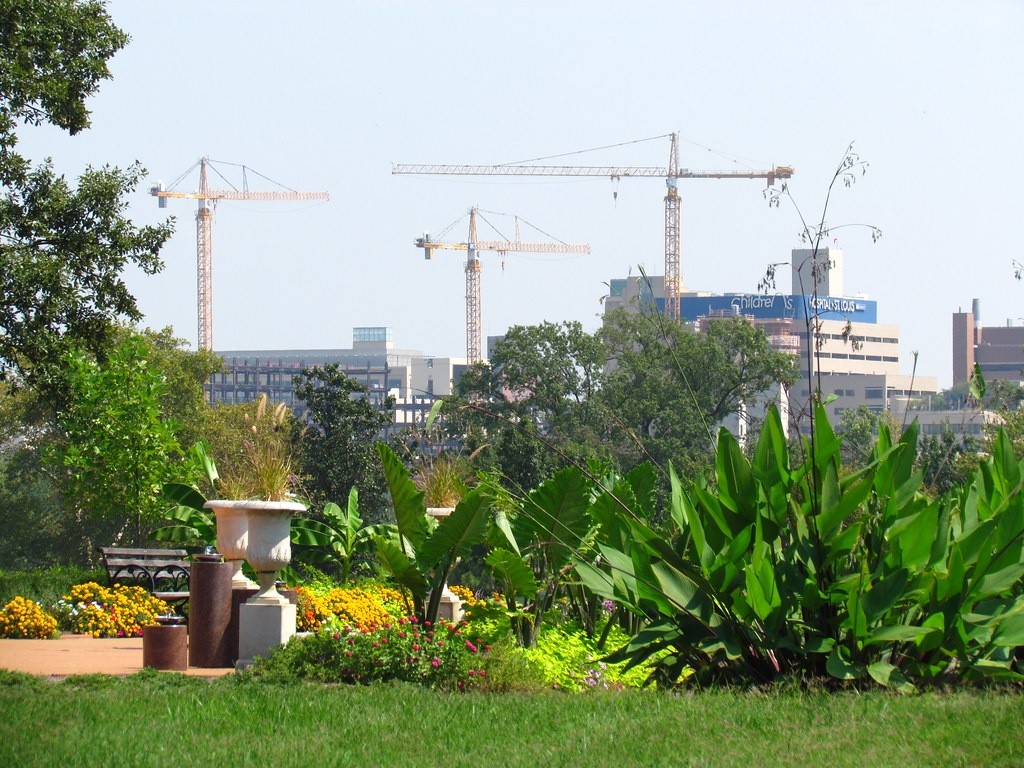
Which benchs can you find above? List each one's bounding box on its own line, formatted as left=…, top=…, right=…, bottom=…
left=97, top=546, right=191, bottom=632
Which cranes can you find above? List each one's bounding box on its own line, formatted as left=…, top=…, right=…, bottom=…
left=412, top=207, right=590, bottom=366
left=149, top=156, right=331, bottom=350
left=390, top=131, right=794, bottom=322
